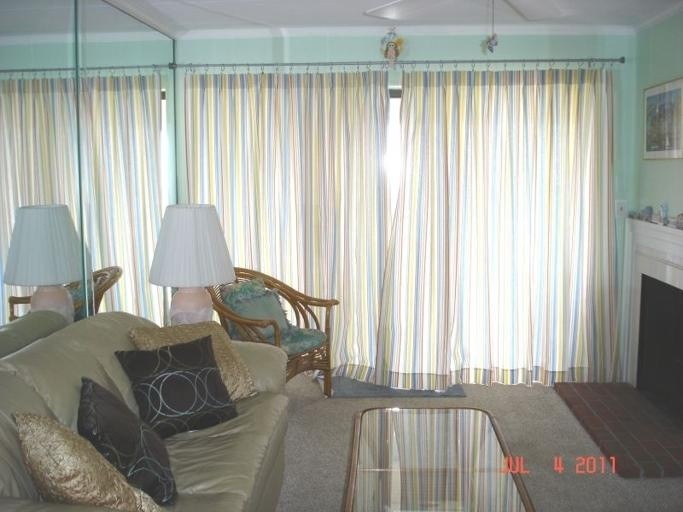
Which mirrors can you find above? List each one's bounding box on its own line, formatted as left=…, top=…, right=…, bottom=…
left=0, top=0, right=177, bottom=329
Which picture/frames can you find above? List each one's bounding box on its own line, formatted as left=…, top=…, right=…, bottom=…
left=642, top=78, right=682, bottom=160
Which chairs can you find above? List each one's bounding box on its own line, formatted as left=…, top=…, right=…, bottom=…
left=204, top=268, right=338, bottom=396
left=8, top=265, right=122, bottom=321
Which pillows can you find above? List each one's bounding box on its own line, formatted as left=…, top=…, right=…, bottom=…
left=115, top=334, right=237, bottom=438
left=11, top=411, right=155, bottom=510
left=129, top=320, right=256, bottom=400
left=79, top=377, right=178, bottom=507
left=230, top=290, right=287, bottom=335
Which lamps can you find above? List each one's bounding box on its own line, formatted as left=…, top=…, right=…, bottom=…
left=2, top=205, right=93, bottom=319
left=149, top=204, right=237, bottom=326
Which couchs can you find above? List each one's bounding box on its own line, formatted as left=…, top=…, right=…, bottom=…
left=0, top=313, right=292, bottom=511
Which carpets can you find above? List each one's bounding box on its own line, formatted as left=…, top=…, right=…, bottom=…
left=333, top=369, right=465, bottom=397
